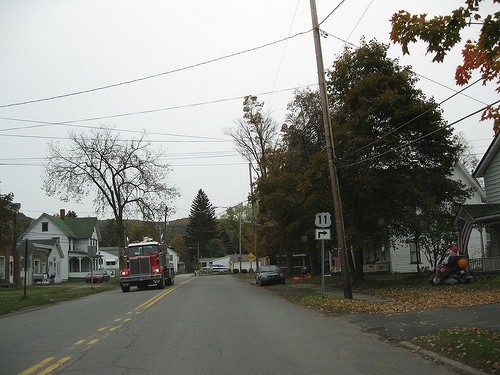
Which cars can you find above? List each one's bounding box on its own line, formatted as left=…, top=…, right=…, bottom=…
left=255, top=265, right=286, bottom=286
left=86, top=270, right=110, bottom=283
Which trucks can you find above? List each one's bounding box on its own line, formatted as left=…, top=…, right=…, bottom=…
left=119, top=233, right=175, bottom=293
